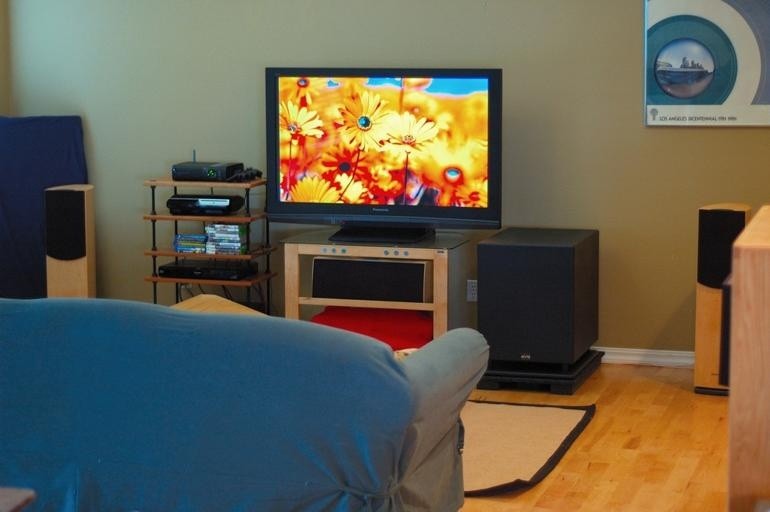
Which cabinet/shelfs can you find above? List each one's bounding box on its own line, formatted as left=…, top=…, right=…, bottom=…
left=143, top=174, right=277, bottom=319
left=280, top=229, right=473, bottom=351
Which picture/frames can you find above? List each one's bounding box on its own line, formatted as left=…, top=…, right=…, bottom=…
left=644, top=1, right=769, bottom=128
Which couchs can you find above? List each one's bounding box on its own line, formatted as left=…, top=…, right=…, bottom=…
left=0, top=296, right=490, bottom=512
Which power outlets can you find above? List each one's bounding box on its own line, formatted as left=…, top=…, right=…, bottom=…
left=466, top=280, right=479, bottom=304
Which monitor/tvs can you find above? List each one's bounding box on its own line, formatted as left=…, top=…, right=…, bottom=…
left=265, top=67, right=503, bottom=244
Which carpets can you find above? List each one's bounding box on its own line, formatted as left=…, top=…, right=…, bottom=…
left=457, top=398, right=597, bottom=497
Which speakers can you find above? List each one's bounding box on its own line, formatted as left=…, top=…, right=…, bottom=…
left=44, top=185, right=96, bottom=298
left=694, top=203, right=753, bottom=396
